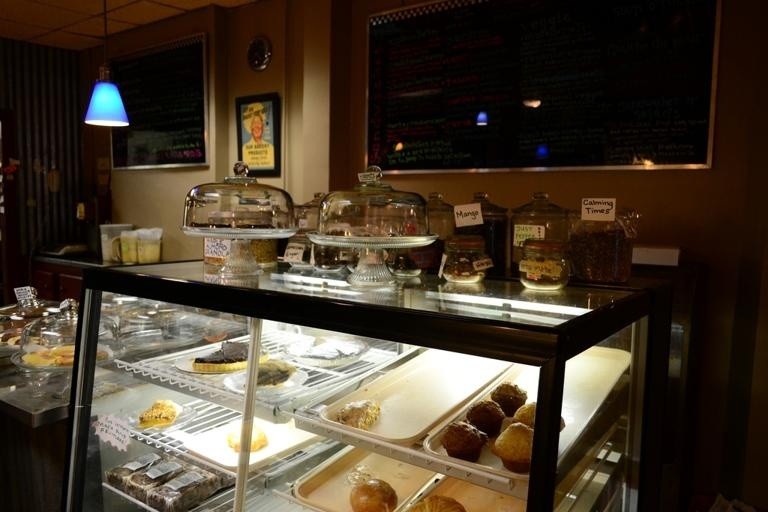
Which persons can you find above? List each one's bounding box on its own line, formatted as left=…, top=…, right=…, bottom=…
left=239, top=104, right=275, bottom=170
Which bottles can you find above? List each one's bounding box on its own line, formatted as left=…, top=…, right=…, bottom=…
left=301, top=191, right=640, bottom=291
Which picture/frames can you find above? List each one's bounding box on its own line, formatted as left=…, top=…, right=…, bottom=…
left=235, top=91, right=281, bottom=178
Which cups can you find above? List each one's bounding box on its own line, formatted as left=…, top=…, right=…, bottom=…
left=136, top=239, right=161, bottom=264
left=100, top=224, right=134, bottom=262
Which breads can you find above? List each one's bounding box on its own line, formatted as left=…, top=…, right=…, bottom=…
left=104, top=450, right=234, bottom=512
left=228, top=427, right=268, bottom=451
left=440, top=381, right=566, bottom=475
left=407, top=495, right=466, bottom=512
left=137, top=401, right=175, bottom=426
left=192, top=341, right=296, bottom=386
left=20, top=346, right=108, bottom=366
left=349, top=479, right=398, bottom=511
left=332, top=399, right=381, bottom=430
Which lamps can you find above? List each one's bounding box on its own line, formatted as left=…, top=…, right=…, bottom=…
left=474, top=109, right=489, bottom=127
left=83, top=0, right=129, bottom=128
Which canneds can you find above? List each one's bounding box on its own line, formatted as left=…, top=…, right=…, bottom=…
left=518, top=238, right=568, bottom=290
left=443, top=233, right=485, bottom=284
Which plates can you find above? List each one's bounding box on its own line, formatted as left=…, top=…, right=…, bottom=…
left=225, top=367, right=307, bottom=395
left=174, top=353, right=236, bottom=375
left=284, top=338, right=367, bottom=366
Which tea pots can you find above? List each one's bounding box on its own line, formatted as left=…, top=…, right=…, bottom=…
left=111, top=229, right=137, bottom=264
